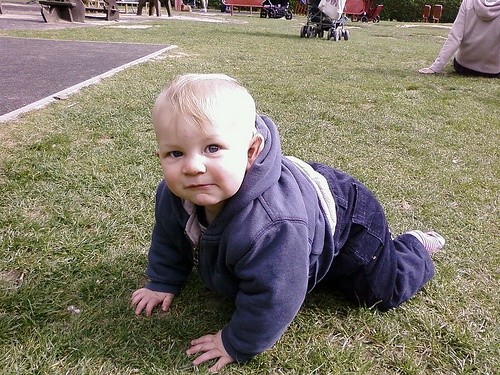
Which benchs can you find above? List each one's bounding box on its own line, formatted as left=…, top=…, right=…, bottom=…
left=38, top=0, right=121, bottom=24
left=222, top=0, right=264, bottom=16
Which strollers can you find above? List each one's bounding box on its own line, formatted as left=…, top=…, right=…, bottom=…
left=298, top=0, right=355, bottom=42
left=260, top=1, right=293, bottom=22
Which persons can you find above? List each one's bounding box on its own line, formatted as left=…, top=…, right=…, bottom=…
left=220, top=3, right=231, bottom=12
left=418, top=0, right=500, bottom=78
left=129, top=74, right=445, bottom=372
left=202, top=0, right=209, bottom=13
